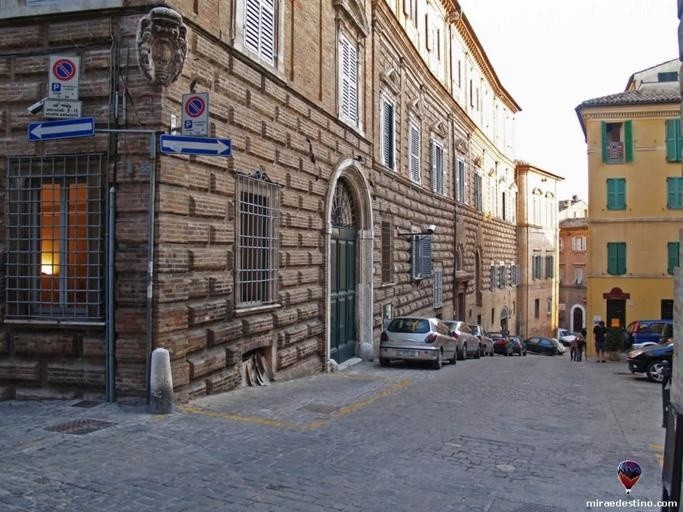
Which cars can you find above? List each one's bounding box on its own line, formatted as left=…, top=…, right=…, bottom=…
left=378, top=316, right=580, bottom=369
left=625, top=337, right=672, bottom=384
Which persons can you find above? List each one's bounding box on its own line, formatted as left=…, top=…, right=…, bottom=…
left=593, top=323, right=609, bottom=362
left=570, top=328, right=586, bottom=362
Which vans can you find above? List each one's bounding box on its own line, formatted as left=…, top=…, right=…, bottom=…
left=627, top=320, right=673, bottom=351
